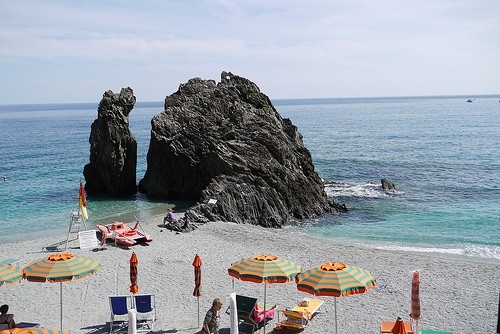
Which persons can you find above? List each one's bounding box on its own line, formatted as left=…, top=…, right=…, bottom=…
left=203, top=298, right=224, bottom=334
left=252, top=303, right=276, bottom=317
left=163, top=208, right=177, bottom=225
left=0, top=305, right=15, bottom=326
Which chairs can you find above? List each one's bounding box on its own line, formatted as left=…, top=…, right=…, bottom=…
left=108, top=293, right=157, bottom=334
left=275, top=298, right=325, bottom=330
left=0, top=322, right=40, bottom=331
left=380, top=320, right=414, bottom=334
left=225, top=295, right=274, bottom=334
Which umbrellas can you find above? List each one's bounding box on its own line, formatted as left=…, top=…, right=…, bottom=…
left=130, top=252, right=139, bottom=295
left=391, top=317, right=406, bottom=334
left=0, top=257, right=24, bottom=287
left=0, top=327, right=72, bottom=334
left=24, top=254, right=102, bottom=330
left=192, top=254, right=203, bottom=328
left=228, top=254, right=303, bottom=334
left=409, top=271, right=422, bottom=334
left=295, top=261, right=376, bottom=334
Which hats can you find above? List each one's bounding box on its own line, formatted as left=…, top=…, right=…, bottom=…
left=167, top=208, right=172, bottom=211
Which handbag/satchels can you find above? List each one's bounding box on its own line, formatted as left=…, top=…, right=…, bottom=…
left=198, top=327, right=207, bottom=334
